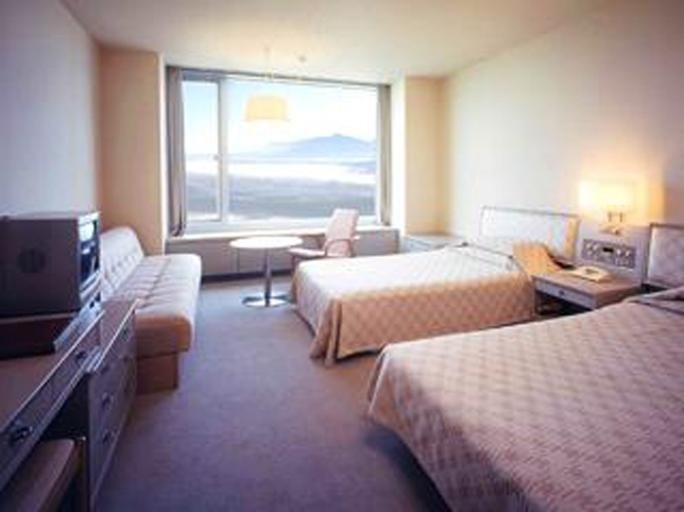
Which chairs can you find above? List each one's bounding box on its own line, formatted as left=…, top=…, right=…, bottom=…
left=286, top=207, right=364, bottom=278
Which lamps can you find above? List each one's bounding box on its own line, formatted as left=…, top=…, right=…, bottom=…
left=595, top=180, right=635, bottom=237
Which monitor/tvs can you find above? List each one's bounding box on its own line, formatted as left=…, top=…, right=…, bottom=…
left=0, top=210, right=104, bottom=314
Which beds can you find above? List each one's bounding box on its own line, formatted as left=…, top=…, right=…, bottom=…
left=291, top=204, right=580, bottom=367
left=369, top=220, right=683, bottom=510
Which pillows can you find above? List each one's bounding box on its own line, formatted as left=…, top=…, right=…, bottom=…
left=459, top=228, right=556, bottom=270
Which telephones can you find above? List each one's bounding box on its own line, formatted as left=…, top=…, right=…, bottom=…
left=575, top=265, right=611, bottom=282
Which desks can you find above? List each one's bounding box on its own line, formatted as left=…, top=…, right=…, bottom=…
left=230, top=234, right=304, bottom=310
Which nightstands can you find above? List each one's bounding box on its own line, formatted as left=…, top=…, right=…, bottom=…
left=403, top=232, right=456, bottom=251
left=528, top=266, right=643, bottom=323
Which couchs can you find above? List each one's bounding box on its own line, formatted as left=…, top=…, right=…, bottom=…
left=98, top=221, right=203, bottom=393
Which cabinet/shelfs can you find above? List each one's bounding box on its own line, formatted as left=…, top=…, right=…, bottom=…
left=0, top=298, right=140, bottom=512
